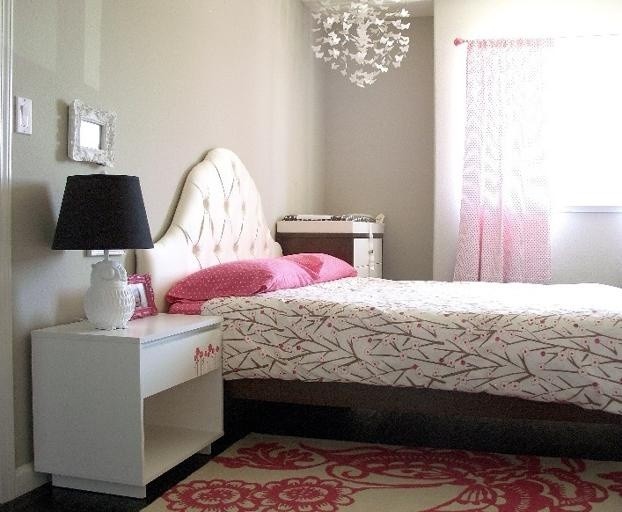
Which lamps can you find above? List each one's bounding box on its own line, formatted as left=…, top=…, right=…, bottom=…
left=50, top=172, right=158, bottom=332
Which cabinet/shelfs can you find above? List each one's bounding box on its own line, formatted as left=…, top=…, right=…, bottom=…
left=276, top=222, right=385, bottom=281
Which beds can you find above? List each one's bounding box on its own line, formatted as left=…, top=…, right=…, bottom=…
left=136, top=149, right=622, bottom=462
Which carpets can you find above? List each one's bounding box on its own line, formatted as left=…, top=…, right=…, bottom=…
left=140, top=431, right=622, bottom=512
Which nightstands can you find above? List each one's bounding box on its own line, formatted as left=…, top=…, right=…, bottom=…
left=29, top=324, right=230, bottom=500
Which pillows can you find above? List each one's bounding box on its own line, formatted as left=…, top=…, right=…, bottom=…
left=166, top=252, right=358, bottom=312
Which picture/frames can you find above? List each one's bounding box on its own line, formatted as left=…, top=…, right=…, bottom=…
left=124, top=273, right=158, bottom=323
left=66, top=99, right=118, bottom=167
